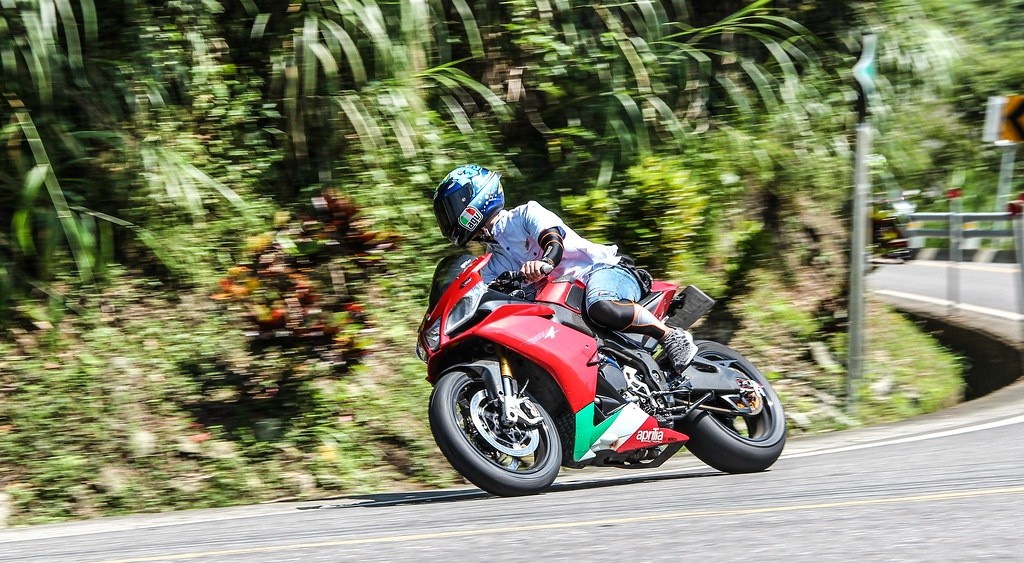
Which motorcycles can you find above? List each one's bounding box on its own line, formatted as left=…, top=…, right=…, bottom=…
left=416, top=249, right=788, bottom=498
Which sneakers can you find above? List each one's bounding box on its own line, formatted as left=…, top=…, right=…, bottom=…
left=660, top=326, right=698, bottom=373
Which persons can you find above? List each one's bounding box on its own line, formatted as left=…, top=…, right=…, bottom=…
left=432, top=164, right=697, bottom=373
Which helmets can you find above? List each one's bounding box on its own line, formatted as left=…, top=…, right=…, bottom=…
left=433, top=165, right=504, bottom=247
